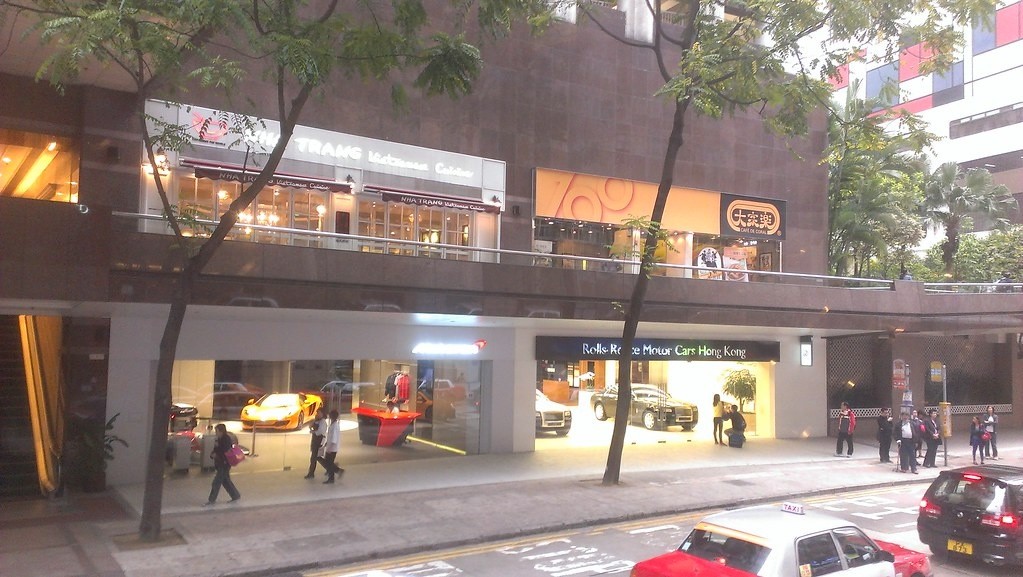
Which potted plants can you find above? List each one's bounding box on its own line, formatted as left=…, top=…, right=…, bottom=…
left=723, top=369, right=756, bottom=431
left=68, top=419, right=127, bottom=495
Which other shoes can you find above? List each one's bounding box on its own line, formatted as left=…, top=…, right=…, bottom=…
left=985, top=456, right=990, bottom=460
left=880, top=459, right=893, bottom=464
left=980, top=462, right=985, bottom=464
left=912, top=471, right=919, bottom=474
left=931, top=465, right=937, bottom=468
left=227, top=496, right=241, bottom=504
left=323, top=477, right=334, bottom=484
left=325, top=470, right=330, bottom=476
left=899, top=468, right=907, bottom=473
left=201, top=500, right=215, bottom=507
left=923, top=464, right=930, bottom=468
left=973, top=461, right=978, bottom=465
left=834, top=452, right=854, bottom=458
left=714, top=441, right=728, bottom=446
left=304, top=472, right=314, bottom=480
left=993, top=456, right=998, bottom=460
left=336, top=468, right=344, bottom=480
left=916, top=460, right=921, bottom=465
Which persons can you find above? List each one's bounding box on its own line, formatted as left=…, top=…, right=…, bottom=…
left=834, top=401, right=856, bottom=457
left=602, top=253, right=623, bottom=272
left=713, top=394, right=728, bottom=446
left=983, top=406, right=999, bottom=459
left=895, top=408, right=942, bottom=474
left=996, top=271, right=1014, bottom=292
left=829, top=534, right=870, bottom=564
left=969, top=416, right=987, bottom=465
left=877, top=407, right=894, bottom=463
left=724, top=404, right=747, bottom=442
left=305, top=407, right=345, bottom=484
left=385, top=401, right=398, bottom=413
left=201, top=424, right=241, bottom=507
left=899, top=269, right=911, bottom=280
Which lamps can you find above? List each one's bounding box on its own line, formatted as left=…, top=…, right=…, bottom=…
left=492, top=195, right=502, bottom=204
left=346, top=174, right=357, bottom=184
left=156, top=146, right=165, bottom=162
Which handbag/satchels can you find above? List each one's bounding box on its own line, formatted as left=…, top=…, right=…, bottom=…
left=224, top=444, right=245, bottom=467
left=980, top=423, right=991, bottom=442
left=722, top=412, right=729, bottom=421
left=937, top=435, right=943, bottom=445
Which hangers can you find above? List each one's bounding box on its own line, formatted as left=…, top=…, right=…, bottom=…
left=396, top=370, right=408, bottom=376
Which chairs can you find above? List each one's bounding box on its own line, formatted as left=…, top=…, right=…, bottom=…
left=949, top=486, right=1023, bottom=511
left=726, top=537, right=836, bottom=562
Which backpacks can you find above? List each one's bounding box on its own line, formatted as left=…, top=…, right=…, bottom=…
left=732, top=412, right=747, bottom=429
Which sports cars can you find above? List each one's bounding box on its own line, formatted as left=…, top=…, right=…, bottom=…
left=240, top=392, right=323, bottom=430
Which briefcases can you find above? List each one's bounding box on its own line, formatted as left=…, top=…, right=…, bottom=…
left=729, top=434, right=743, bottom=448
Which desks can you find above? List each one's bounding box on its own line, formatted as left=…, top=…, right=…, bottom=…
left=350, top=406, right=421, bottom=447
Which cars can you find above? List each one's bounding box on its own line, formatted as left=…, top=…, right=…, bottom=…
left=168, top=403, right=198, bottom=432
left=168, top=376, right=482, bottom=427
left=591, top=383, right=698, bottom=430
left=917, top=464, right=1023, bottom=568
left=535, top=389, right=571, bottom=436
left=630, top=501, right=932, bottom=577
